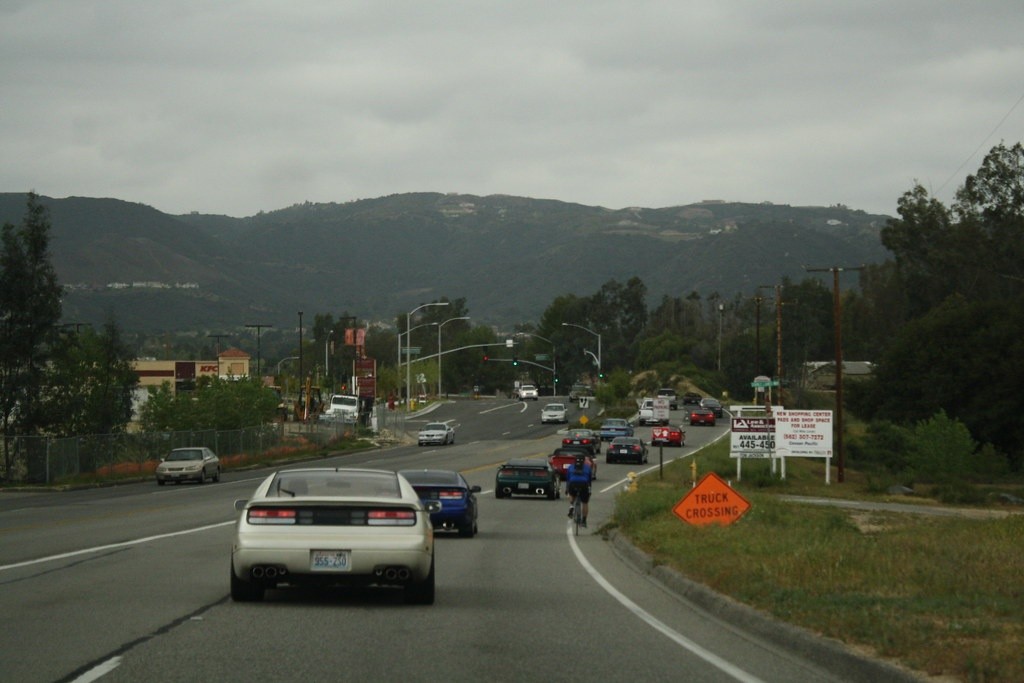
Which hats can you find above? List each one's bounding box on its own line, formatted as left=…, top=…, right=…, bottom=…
left=576, top=455, right=585, bottom=462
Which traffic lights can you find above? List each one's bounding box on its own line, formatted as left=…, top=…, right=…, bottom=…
left=599, top=370, right=603, bottom=378
left=555, top=373, right=559, bottom=383
left=513, top=357, right=517, bottom=366
left=341, top=386, right=346, bottom=391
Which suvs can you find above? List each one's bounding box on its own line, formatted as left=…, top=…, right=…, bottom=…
left=518, top=385, right=539, bottom=401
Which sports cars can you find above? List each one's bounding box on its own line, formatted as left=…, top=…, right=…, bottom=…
left=228, top=467, right=436, bottom=608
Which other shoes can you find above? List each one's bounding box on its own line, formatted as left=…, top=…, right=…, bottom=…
left=582, top=522, right=586, bottom=527
left=568, top=505, right=574, bottom=518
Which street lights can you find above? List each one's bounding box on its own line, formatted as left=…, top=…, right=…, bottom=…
left=512, top=323, right=601, bottom=397
left=278, top=356, right=300, bottom=375
left=398, top=302, right=471, bottom=414
left=325, top=330, right=333, bottom=376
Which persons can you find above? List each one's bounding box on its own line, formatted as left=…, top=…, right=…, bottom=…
left=565, top=454, right=592, bottom=527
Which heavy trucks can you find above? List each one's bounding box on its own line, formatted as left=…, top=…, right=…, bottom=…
left=325, top=394, right=361, bottom=425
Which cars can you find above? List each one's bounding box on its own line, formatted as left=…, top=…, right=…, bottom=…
left=548, top=447, right=597, bottom=482
left=600, top=418, right=634, bottom=442
left=399, top=469, right=482, bottom=538
left=495, top=457, right=562, bottom=500
left=418, top=423, right=455, bottom=446
left=606, top=437, right=649, bottom=464
left=562, top=429, right=603, bottom=455
left=569, top=391, right=586, bottom=402
left=638, top=388, right=723, bottom=426
left=541, top=403, right=568, bottom=424
left=156, top=447, right=222, bottom=484
left=653, top=426, right=686, bottom=446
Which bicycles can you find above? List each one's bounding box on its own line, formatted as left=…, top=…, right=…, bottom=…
left=572, top=487, right=583, bottom=535
